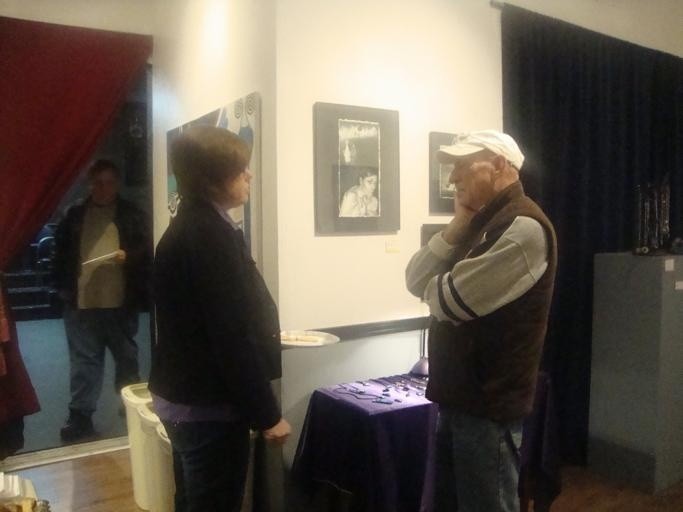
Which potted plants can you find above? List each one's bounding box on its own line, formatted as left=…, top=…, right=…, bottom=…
left=429, top=131, right=458, bottom=213
left=311, top=101, right=400, bottom=234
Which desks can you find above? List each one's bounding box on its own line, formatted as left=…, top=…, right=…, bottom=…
left=290, top=373, right=563, bottom=512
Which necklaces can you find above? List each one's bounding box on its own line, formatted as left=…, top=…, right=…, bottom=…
left=333, top=374, right=429, bottom=404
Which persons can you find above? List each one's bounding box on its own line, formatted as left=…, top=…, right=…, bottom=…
left=49, top=159, right=154, bottom=441
left=146, top=124, right=293, bottom=512
left=339, top=169, right=380, bottom=218
left=403, top=129, right=559, bottom=512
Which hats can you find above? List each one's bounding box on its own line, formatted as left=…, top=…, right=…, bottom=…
left=435, top=128, right=525, bottom=171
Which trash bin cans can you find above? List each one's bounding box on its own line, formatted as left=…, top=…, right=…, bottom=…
left=120, top=382, right=260, bottom=511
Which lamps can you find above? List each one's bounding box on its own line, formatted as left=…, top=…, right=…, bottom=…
left=409, top=319, right=430, bottom=377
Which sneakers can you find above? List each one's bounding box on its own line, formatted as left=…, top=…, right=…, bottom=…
left=60, top=417, right=93, bottom=440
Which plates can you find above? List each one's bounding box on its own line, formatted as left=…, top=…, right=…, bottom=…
left=80, top=252, right=119, bottom=267
left=280, top=330, right=340, bottom=347
